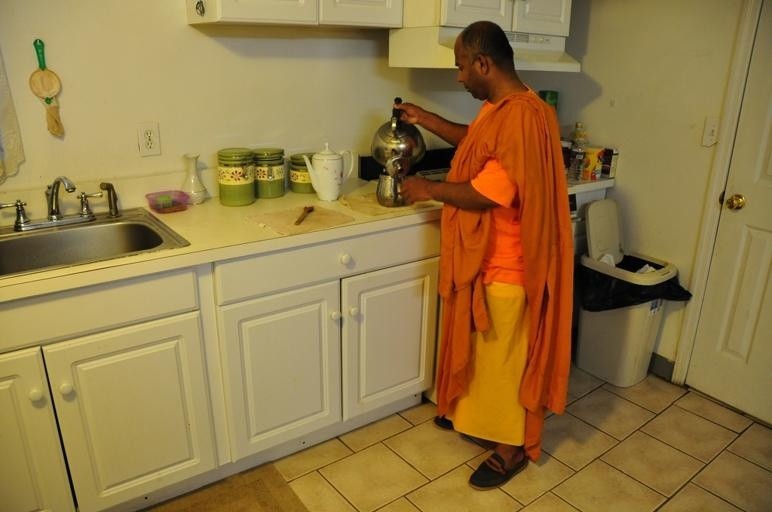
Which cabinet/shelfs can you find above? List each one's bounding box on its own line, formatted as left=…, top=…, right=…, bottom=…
left=0, top=259, right=231, bottom=512
left=183, top=1, right=404, bottom=30
left=389, top=0, right=582, bottom=75
left=205, top=216, right=443, bottom=476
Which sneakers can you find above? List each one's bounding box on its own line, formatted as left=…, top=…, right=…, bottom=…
left=468, top=450, right=528, bottom=491
left=432, top=415, right=454, bottom=431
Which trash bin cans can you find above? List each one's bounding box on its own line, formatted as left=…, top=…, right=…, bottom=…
left=573, top=199, right=678, bottom=388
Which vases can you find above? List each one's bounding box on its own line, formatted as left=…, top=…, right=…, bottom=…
left=180, top=153, right=207, bottom=205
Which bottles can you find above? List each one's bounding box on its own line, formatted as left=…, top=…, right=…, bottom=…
left=574, top=122, right=584, bottom=146
left=568, top=138, right=586, bottom=183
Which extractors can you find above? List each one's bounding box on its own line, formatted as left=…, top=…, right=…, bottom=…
left=389, top=27, right=581, bottom=72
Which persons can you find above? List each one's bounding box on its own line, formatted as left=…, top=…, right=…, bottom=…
left=393, top=21, right=574, bottom=490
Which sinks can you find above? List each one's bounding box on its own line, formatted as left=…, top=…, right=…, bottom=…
left=0, top=206, right=192, bottom=280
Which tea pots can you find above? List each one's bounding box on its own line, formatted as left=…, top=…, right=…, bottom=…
left=304, top=143, right=354, bottom=200
left=370, top=97, right=426, bottom=173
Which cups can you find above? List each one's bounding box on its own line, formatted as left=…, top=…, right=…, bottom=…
left=376, top=155, right=410, bottom=207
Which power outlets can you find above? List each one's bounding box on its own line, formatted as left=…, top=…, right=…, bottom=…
left=137, top=121, right=161, bottom=156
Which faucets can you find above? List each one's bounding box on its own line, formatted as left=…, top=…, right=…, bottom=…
left=46, top=175, right=75, bottom=220
